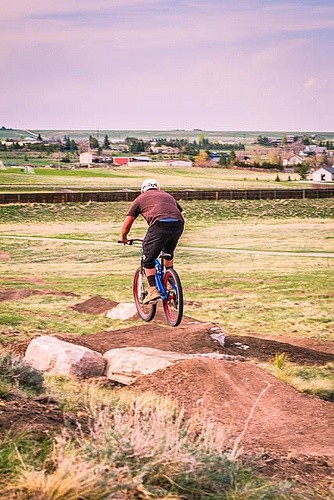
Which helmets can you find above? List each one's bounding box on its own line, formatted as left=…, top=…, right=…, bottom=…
left=141, top=179, right=160, bottom=194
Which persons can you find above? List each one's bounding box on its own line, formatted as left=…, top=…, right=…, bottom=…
left=121, top=178, right=185, bottom=305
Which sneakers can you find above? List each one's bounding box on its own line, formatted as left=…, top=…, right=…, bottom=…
left=167, top=278, right=175, bottom=289
left=142, top=287, right=161, bottom=304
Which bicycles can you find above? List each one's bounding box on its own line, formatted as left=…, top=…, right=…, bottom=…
left=117, top=238, right=184, bottom=327
